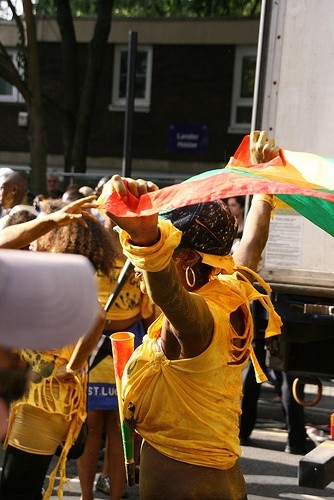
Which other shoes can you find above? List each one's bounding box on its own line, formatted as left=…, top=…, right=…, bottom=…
left=96, top=475, right=111, bottom=496
left=285, top=438, right=320, bottom=454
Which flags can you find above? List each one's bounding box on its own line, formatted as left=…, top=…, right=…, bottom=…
left=96, top=136, right=334, bottom=239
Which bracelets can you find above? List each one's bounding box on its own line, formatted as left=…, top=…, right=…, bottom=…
left=117, top=220, right=181, bottom=272
left=252, top=194, right=277, bottom=209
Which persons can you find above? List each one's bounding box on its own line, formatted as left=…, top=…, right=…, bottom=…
left=104, top=130, right=282, bottom=500
left=239, top=367, right=317, bottom=455
left=0, top=166, right=157, bottom=500
left=227, top=196, right=245, bottom=252
